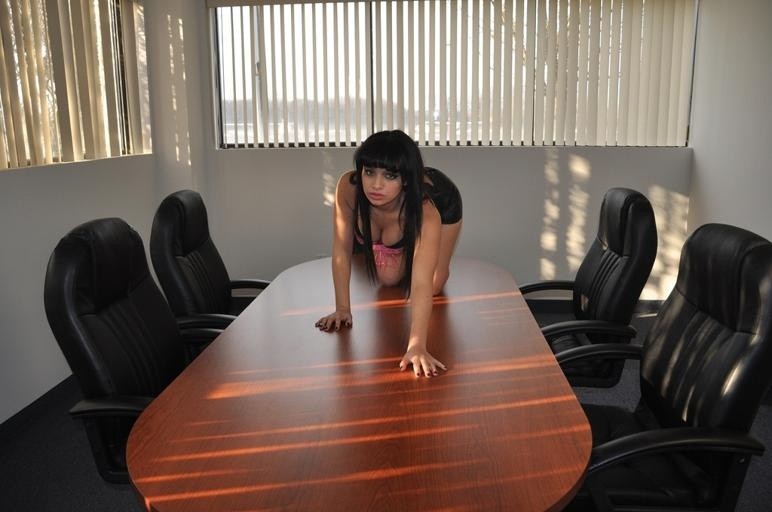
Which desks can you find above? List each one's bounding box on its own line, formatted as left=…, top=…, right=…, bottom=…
left=126, top=255, right=593, bottom=512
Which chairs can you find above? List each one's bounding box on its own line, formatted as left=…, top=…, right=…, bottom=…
left=554, top=224, right=772, bottom=512
left=45, top=218, right=223, bottom=484
left=519, top=187, right=658, bottom=388
left=150, top=190, right=271, bottom=328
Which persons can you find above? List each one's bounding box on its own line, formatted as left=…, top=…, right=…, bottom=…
left=315, top=129, right=463, bottom=378
